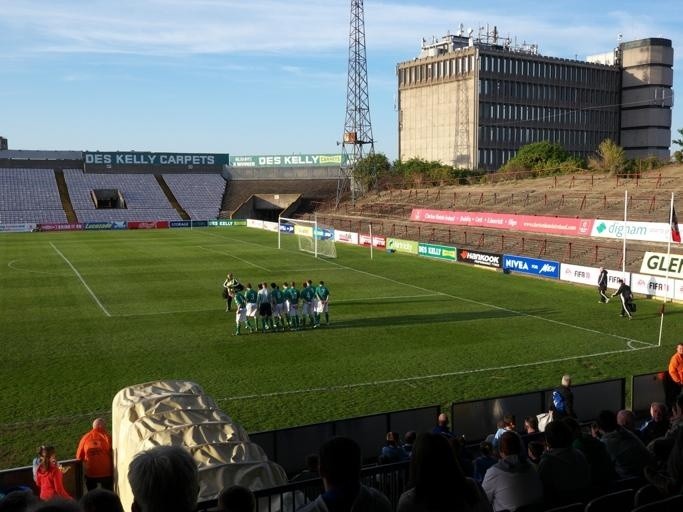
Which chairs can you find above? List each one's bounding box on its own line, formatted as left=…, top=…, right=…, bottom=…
left=584, top=488, right=632, bottom=511
left=629, top=494, right=682, bottom=512
left=633, top=484, right=664, bottom=504
left=545, top=500, right=583, bottom=511
left=0, top=165, right=226, bottom=223
left=610, top=473, right=640, bottom=489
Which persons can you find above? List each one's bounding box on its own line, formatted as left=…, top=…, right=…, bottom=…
left=610, top=279, right=634, bottom=321
left=597, top=266, right=610, bottom=304
left=222, top=271, right=330, bottom=335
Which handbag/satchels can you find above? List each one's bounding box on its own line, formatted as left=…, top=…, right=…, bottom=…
left=551, top=390, right=565, bottom=415
left=622, top=302, right=636, bottom=312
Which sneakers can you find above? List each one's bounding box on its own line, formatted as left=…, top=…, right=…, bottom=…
left=225, top=305, right=332, bottom=336
left=619, top=314, right=633, bottom=321
left=598, top=298, right=610, bottom=304
left=643, top=464, right=681, bottom=498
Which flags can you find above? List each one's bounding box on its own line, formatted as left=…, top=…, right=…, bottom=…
left=668, top=198, right=681, bottom=243
